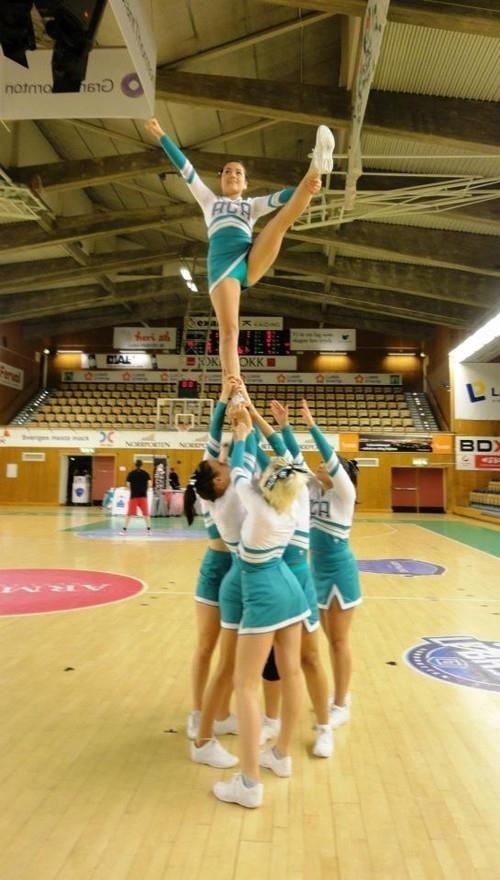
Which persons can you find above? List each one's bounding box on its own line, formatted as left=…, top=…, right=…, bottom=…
left=169, top=468, right=181, bottom=490
left=145, top=118, right=337, bottom=407
left=182, top=369, right=333, bottom=809
left=118, top=460, right=154, bottom=536
left=268, top=399, right=365, bottom=727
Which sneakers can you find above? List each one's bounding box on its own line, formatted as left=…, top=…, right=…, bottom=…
left=258, top=714, right=282, bottom=745
left=307, top=121, right=337, bottom=179
left=119, top=529, right=154, bottom=535
left=210, top=773, right=263, bottom=811
left=310, top=691, right=354, bottom=728
left=312, top=722, right=334, bottom=759
left=189, top=737, right=237, bottom=771
left=183, top=710, right=240, bottom=738
left=258, top=747, right=293, bottom=779
